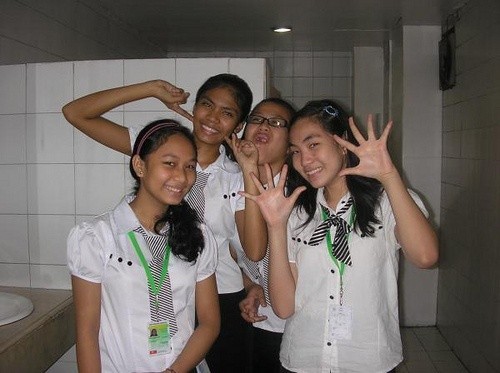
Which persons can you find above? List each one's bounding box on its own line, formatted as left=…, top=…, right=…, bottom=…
left=238, top=99, right=438, bottom=373
left=65, top=120, right=220, bottom=373
left=228, top=97, right=297, bottom=373
left=151, top=329, right=157, bottom=337
left=63, top=73, right=253, bottom=373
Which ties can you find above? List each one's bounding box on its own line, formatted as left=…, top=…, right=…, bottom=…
left=242, top=239, right=271, bottom=307
left=134, top=226, right=178, bottom=339
left=185, top=171, right=211, bottom=222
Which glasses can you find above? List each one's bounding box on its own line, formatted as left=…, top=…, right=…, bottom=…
left=247, top=115, right=290, bottom=132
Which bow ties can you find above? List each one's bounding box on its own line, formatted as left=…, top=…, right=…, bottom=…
left=308, top=197, right=354, bottom=266
